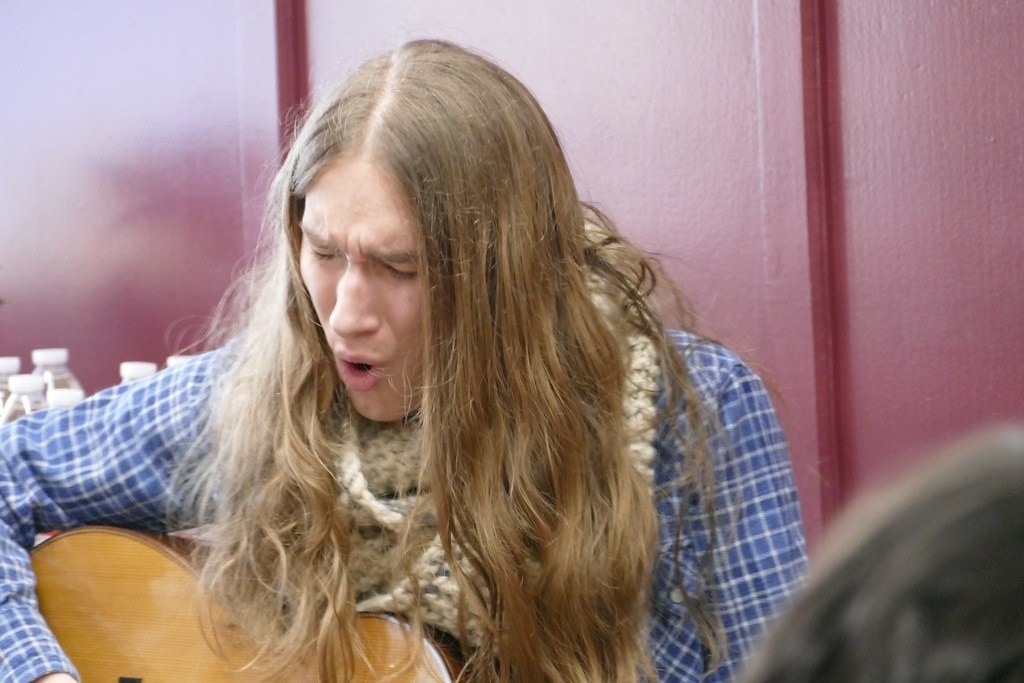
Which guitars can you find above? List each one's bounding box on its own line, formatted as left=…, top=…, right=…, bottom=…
left=29, top=522, right=471, bottom=683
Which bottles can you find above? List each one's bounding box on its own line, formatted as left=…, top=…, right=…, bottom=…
left=1, top=347, right=202, bottom=424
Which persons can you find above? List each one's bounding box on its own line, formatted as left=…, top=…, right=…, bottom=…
left=737, top=419, right=1024, bottom=683
left=0, top=39, right=810, bottom=683
